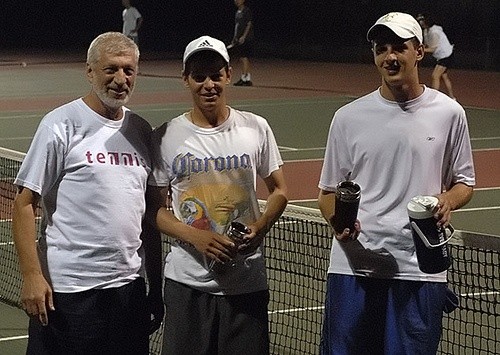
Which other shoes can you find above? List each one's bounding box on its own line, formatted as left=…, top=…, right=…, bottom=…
left=233, top=79, right=252, bottom=86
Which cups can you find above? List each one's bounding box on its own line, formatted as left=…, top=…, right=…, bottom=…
left=334, top=181, right=360, bottom=234
left=208, top=221, right=254, bottom=277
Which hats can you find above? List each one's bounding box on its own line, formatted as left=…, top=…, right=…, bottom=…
left=366, top=12, right=423, bottom=46
left=183, top=35, right=230, bottom=67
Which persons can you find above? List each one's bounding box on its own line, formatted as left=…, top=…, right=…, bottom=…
left=10, top=31, right=162, bottom=355
left=318, top=12, right=475, bottom=355
left=231, top=0, right=253, bottom=86
left=416, top=12, right=458, bottom=101
left=151, top=36, right=290, bottom=355
left=122, top=0, right=143, bottom=75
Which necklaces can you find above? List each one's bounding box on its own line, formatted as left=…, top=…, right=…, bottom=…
left=190, top=111, right=195, bottom=123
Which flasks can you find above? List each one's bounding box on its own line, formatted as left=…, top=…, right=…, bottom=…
left=407, top=195, right=452, bottom=274
